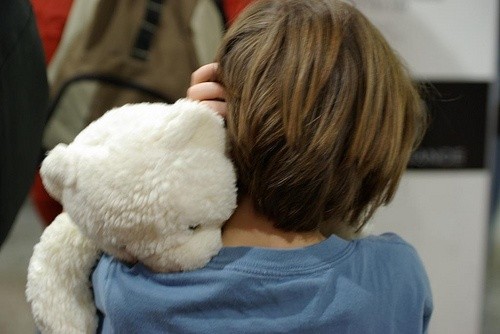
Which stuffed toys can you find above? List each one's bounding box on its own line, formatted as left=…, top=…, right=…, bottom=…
left=26, top=98, right=238, bottom=334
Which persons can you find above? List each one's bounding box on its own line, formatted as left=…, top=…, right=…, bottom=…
left=90, top=1, right=433, bottom=334
left=31, top=2, right=252, bottom=230
